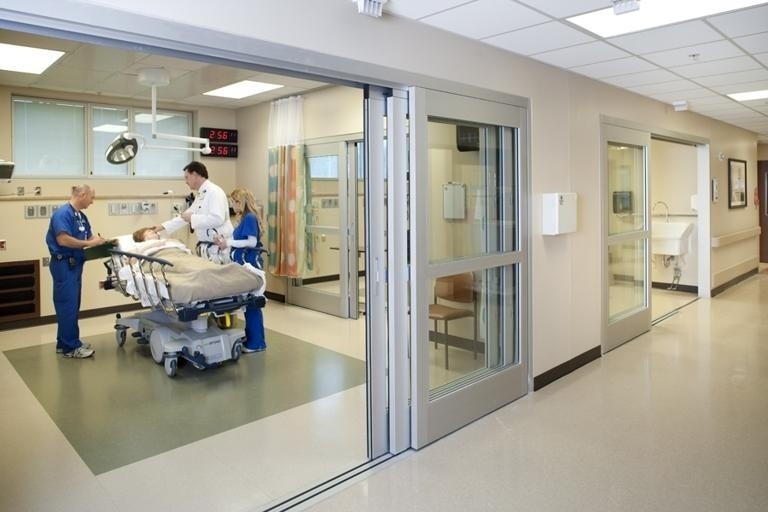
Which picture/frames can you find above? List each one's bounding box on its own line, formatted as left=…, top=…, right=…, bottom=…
left=727, top=157, right=748, bottom=210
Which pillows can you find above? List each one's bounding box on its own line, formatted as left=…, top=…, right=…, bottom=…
left=114, top=233, right=138, bottom=250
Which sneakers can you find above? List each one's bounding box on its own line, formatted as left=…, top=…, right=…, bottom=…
left=57, top=343, right=95, bottom=358
left=240, top=346, right=266, bottom=353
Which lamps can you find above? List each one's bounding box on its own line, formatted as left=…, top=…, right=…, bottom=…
left=102, top=69, right=211, bottom=167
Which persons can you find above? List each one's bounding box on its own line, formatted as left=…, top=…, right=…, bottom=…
left=214, top=186, right=269, bottom=353
left=43, top=182, right=121, bottom=360
left=122, top=227, right=162, bottom=266
left=151, top=159, right=234, bottom=265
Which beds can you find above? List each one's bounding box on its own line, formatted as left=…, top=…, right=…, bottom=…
left=99, top=228, right=270, bottom=377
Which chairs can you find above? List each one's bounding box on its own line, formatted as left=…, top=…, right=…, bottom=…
left=429, top=272, right=480, bottom=371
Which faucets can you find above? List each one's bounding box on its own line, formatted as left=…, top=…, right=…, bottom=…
left=652, top=201, right=669, bottom=223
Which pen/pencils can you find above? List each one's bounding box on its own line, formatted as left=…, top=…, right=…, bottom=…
left=98, top=233, right=102, bottom=238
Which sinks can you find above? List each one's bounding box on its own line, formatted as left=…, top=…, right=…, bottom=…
left=651, top=222, right=693, bottom=256
